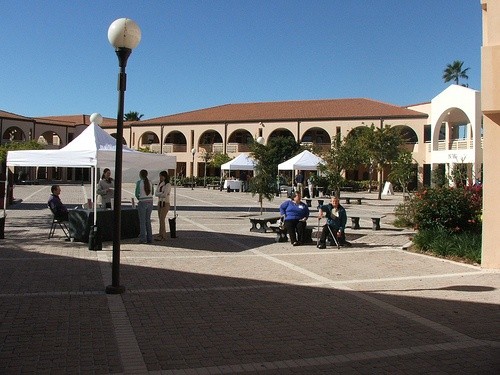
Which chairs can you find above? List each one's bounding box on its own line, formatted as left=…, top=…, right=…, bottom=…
left=47, top=206, right=69, bottom=239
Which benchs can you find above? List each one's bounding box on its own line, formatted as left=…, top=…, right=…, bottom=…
left=302, top=198, right=333, bottom=207
left=340, top=197, right=365, bottom=205
left=249, top=216, right=284, bottom=233
left=346, top=214, right=386, bottom=230
left=207, top=185, right=220, bottom=190
left=270, top=223, right=345, bottom=245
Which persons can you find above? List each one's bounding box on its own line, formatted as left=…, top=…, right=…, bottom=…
left=96, top=168, right=114, bottom=208
left=280, top=191, right=309, bottom=245
left=135, top=169, right=154, bottom=243
left=47, top=185, right=69, bottom=222
left=317, top=197, right=347, bottom=249
left=309, top=172, right=319, bottom=198
left=295, top=172, right=304, bottom=198
left=154, top=171, right=171, bottom=241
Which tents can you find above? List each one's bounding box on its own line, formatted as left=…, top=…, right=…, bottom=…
left=278, top=150, right=331, bottom=198
left=220, top=154, right=258, bottom=193
left=3, top=121, right=177, bottom=251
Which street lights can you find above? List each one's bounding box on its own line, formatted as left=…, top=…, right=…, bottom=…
left=90, top=113, right=102, bottom=212
left=107, top=17, right=140, bottom=293
left=257, top=137, right=265, bottom=201
left=191, top=148, right=195, bottom=184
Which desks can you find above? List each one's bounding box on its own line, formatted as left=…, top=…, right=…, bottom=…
left=67, top=206, right=141, bottom=242
left=287, top=187, right=317, bottom=198
left=224, top=180, right=240, bottom=192
